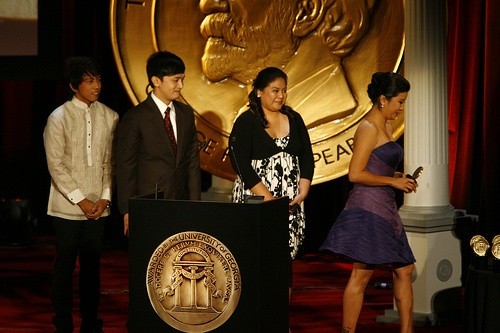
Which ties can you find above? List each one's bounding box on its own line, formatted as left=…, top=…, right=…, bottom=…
left=162, top=108, right=178, bottom=150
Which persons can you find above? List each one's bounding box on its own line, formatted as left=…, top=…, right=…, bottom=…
left=43, top=57, right=120, bottom=333
left=228, top=66, right=315, bottom=333
left=114, top=51, right=201, bottom=236
left=318, top=72, right=419, bottom=333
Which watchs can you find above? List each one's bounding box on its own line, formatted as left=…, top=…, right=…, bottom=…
left=107, top=202, right=109, bottom=208
